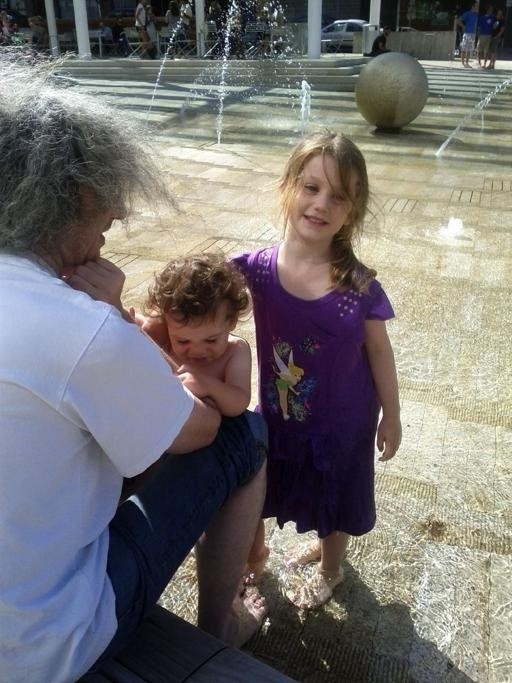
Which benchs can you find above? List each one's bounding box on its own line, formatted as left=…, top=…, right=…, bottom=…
left=75, top=603, right=298, bottom=682
left=90, top=36, right=264, bottom=58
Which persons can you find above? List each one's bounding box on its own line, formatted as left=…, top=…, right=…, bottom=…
left=165, top=0, right=192, bottom=56
left=372, top=25, right=394, bottom=57
left=1, top=77, right=271, bottom=683
left=457, top=1, right=505, bottom=70
left=95, top=0, right=159, bottom=58
left=204, top=0, right=271, bottom=60
left=124, top=250, right=271, bottom=598
left=142, top=129, right=403, bottom=609
left=0, top=10, right=49, bottom=45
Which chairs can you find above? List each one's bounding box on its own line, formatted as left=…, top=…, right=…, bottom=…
left=326, top=24, right=348, bottom=52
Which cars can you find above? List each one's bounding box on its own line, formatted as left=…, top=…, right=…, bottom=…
left=321, top=19, right=418, bottom=53
